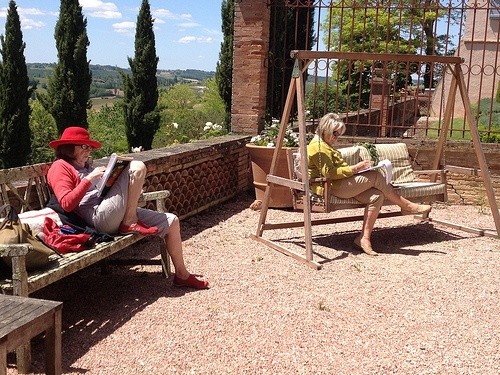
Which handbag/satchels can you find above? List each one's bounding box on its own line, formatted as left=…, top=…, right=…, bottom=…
left=0, top=204, right=54, bottom=269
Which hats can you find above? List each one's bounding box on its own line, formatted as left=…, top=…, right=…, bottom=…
left=50, top=126, right=101, bottom=150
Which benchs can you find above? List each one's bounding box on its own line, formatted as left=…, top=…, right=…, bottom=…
left=0, top=155, right=176, bottom=375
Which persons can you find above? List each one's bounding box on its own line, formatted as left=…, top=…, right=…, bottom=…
left=47, top=127, right=210, bottom=289
left=308, top=113, right=431, bottom=256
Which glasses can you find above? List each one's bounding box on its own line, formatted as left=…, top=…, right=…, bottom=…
left=75, top=144, right=93, bottom=151
left=333, top=131, right=340, bottom=136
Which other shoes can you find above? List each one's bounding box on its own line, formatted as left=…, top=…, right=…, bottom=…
left=401, top=203, right=432, bottom=215
left=354, top=236, right=378, bottom=256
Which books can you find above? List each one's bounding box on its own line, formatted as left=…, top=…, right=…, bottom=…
left=96, top=153, right=133, bottom=198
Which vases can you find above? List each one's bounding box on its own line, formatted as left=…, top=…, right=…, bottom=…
left=246, top=142, right=300, bottom=208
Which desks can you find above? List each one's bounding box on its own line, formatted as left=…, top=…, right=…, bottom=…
left=0, top=292, right=64, bottom=375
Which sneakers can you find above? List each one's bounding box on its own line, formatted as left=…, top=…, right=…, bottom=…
left=175, top=273, right=208, bottom=290
left=119, top=219, right=158, bottom=236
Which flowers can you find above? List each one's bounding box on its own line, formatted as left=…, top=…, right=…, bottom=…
left=245, top=117, right=307, bottom=147
